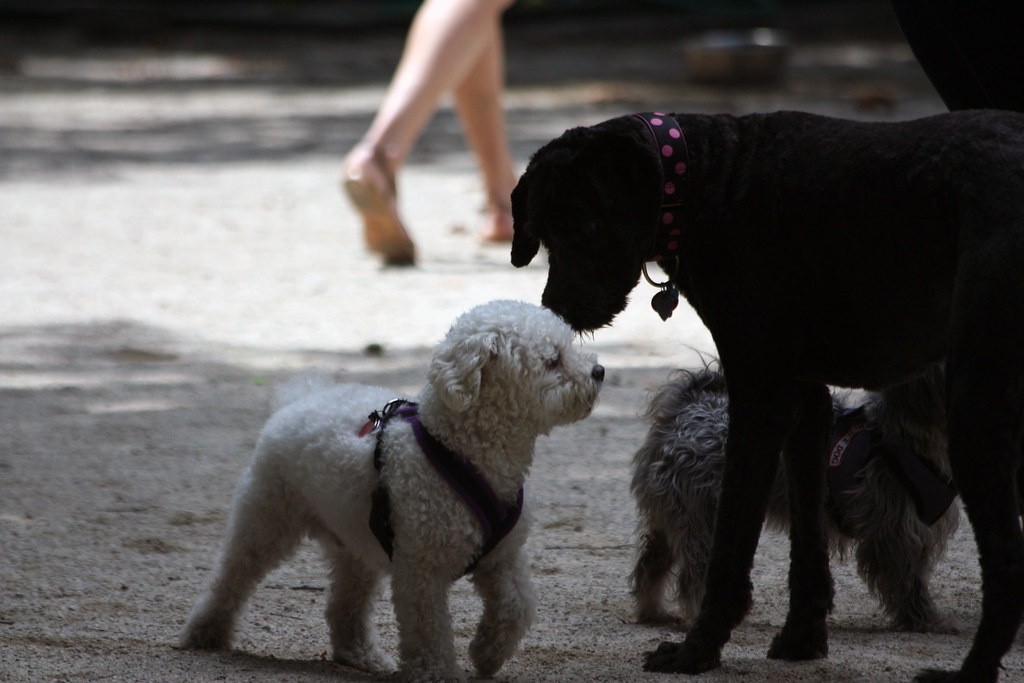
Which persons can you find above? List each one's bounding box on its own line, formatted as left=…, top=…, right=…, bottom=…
left=888, top=1, right=1024, bottom=115
left=340, top=1, right=517, bottom=266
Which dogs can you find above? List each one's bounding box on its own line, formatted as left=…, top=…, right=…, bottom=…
left=508, top=99, right=1023, bottom=683
left=176, top=299, right=607, bottom=683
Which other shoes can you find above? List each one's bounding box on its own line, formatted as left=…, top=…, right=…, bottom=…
left=341, top=142, right=412, bottom=268
